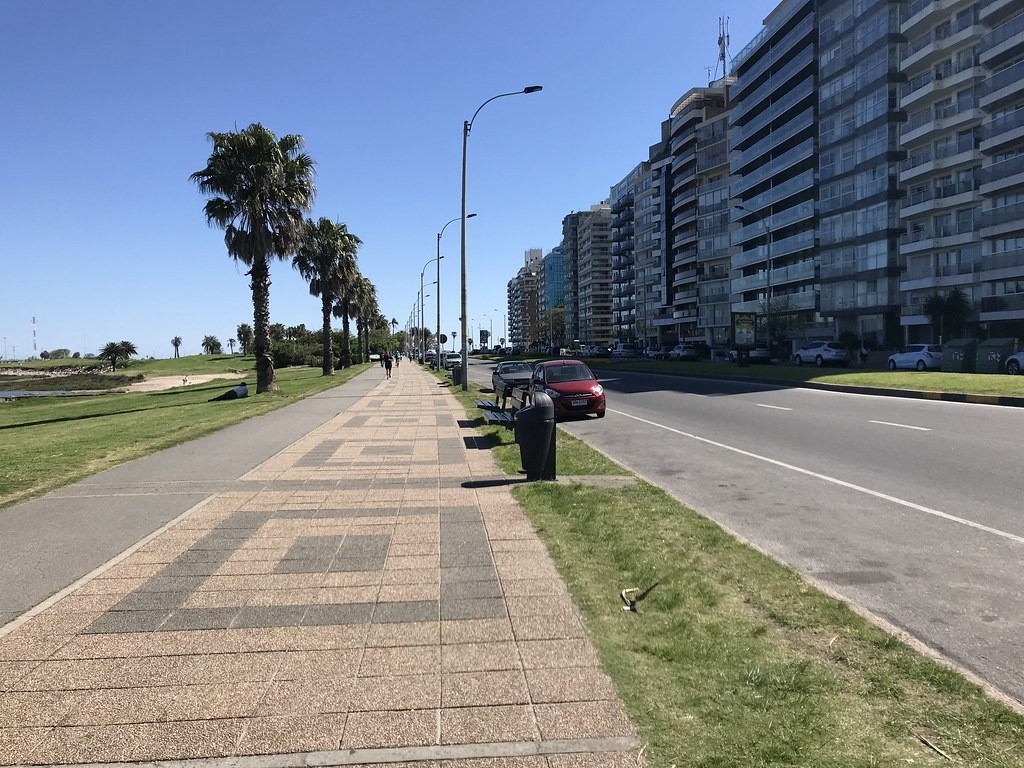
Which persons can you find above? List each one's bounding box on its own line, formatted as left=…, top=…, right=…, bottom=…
left=182, top=375, right=189, bottom=385
left=208, top=382, right=248, bottom=402
left=380, top=351, right=403, bottom=379
left=408, top=351, right=413, bottom=363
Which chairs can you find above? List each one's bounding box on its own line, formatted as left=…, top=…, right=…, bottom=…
left=561, top=367, right=572, bottom=373
left=504, top=367, right=509, bottom=373
left=547, top=370, right=554, bottom=379
left=516, top=365, right=523, bottom=370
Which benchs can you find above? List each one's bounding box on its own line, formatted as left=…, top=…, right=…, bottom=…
left=483, top=387, right=526, bottom=444
left=475, top=382, right=507, bottom=414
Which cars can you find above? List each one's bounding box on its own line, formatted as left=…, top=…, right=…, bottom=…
left=491, top=361, right=533, bottom=396
left=528, top=359, right=606, bottom=422
left=1004, top=351, right=1024, bottom=375
left=413, top=343, right=701, bottom=371
left=729, top=342, right=772, bottom=363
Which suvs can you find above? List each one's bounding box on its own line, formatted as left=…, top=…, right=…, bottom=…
left=793, top=340, right=850, bottom=368
left=887, top=344, right=943, bottom=372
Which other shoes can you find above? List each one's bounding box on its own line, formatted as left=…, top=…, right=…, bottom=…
left=387, top=376, right=388, bottom=379
left=389, top=374, right=391, bottom=377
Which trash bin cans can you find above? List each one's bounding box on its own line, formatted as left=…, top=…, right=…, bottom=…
left=453, top=364, right=461, bottom=386
left=941, top=338, right=977, bottom=372
left=976, top=337, right=1016, bottom=373
left=737, top=349, right=750, bottom=367
left=515, top=392, right=556, bottom=481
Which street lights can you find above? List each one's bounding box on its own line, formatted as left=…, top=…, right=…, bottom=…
left=612, top=253, right=647, bottom=358
left=437, top=213, right=478, bottom=372
left=421, top=256, right=445, bottom=366
left=461, top=84, right=545, bottom=393
left=540, top=294, right=553, bottom=357
left=483, top=314, right=493, bottom=349
left=567, top=277, right=588, bottom=346
left=413, top=295, right=430, bottom=360
left=494, top=308, right=506, bottom=349
left=417, top=282, right=436, bottom=361
left=472, top=319, right=481, bottom=349
left=734, top=205, right=771, bottom=344
